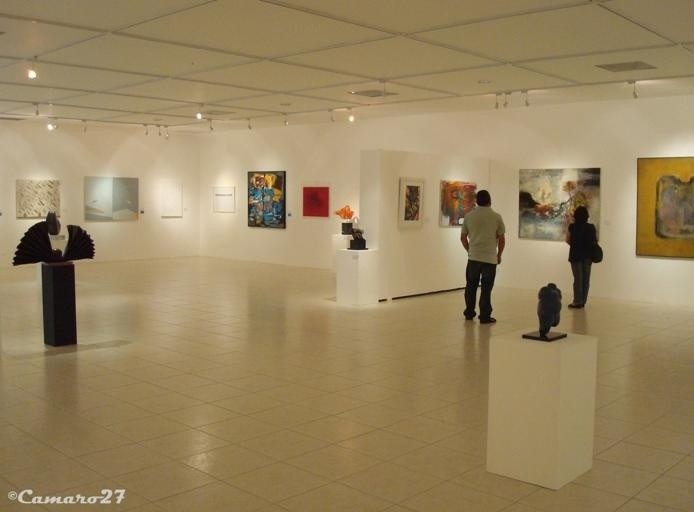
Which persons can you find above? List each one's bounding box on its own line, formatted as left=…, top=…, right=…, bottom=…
left=564, top=206, right=597, bottom=308
left=459, top=191, right=505, bottom=323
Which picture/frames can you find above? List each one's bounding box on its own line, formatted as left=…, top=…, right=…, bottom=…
left=396, top=176, right=425, bottom=230
left=246, top=170, right=288, bottom=229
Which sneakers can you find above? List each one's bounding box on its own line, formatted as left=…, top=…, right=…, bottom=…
left=464, top=314, right=475, bottom=321
left=479, top=317, right=497, bottom=324
left=568, top=303, right=585, bottom=308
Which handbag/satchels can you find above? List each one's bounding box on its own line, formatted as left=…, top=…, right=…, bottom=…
left=591, top=238, right=604, bottom=263
